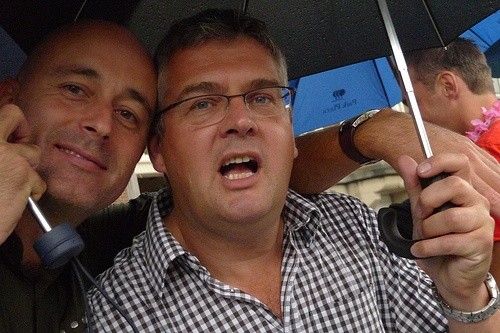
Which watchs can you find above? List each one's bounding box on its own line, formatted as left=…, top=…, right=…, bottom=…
left=338, top=109, right=383, bottom=165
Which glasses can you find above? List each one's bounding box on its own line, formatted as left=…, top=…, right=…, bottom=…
left=154, top=85, right=296, bottom=132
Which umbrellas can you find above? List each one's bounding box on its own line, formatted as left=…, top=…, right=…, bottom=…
left=0, top=0, right=500, bottom=264
left=27, top=196, right=86, bottom=265
left=280, top=10, right=500, bottom=138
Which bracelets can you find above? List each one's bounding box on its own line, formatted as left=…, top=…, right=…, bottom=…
left=431, top=271, right=500, bottom=324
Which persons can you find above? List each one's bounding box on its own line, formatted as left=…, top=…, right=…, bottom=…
left=0, top=21, right=499, bottom=333
left=397, top=35, right=500, bottom=291
left=82, top=7, right=500, bottom=332
